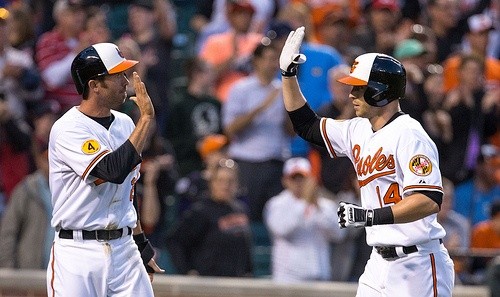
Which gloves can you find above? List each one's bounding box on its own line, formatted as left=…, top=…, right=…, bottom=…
left=336, top=201, right=374, bottom=229
left=279, top=26, right=307, bottom=76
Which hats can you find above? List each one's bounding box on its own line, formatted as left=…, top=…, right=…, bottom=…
left=370, top=0, right=400, bottom=13
left=71, top=42, right=140, bottom=95
left=392, top=39, right=425, bottom=61
left=336, top=52, right=407, bottom=108
left=197, top=135, right=230, bottom=160
left=282, top=157, right=311, bottom=179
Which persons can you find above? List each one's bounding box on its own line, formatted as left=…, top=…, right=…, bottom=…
left=46, top=42, right=165, bottom=297
left=279, top=27, right=456, bottom=297
left=0, top=0, right=500, bottom=281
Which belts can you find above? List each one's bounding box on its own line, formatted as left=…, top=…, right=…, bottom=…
left=58, top=226, right=132, bottom=240
left=375, top=239, right=444, bottom=258
